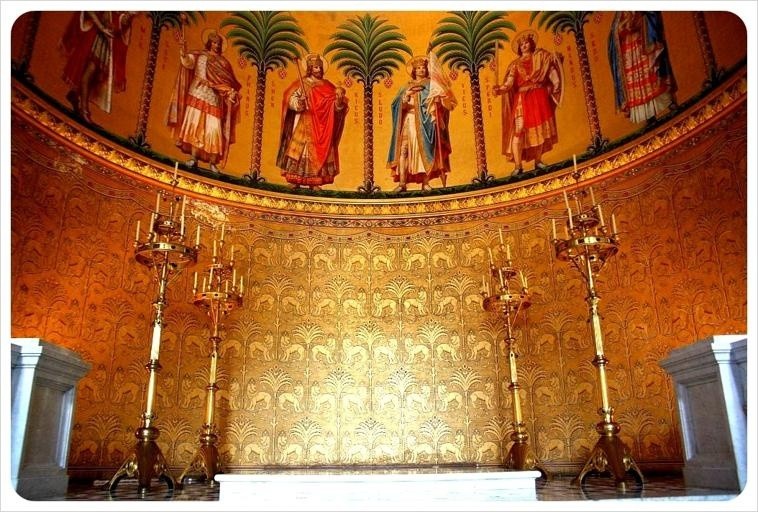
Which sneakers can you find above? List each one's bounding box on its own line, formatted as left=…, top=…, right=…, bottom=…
left=66, top=91, right=92, bottom=124
left=209, top=164, right=220, bottom=174
left=422, top=185, right=433, bottom=193
left=288, top=183, right=300, bottom=190
left=309, top=185, right=321, bottom=191
left=510, top=167, right=524, bottom=177
left=393, top=185, right=407, bottom=194
left=535, top=162, right=549, bottom=170
left=186, top=157, right=198, bottom=167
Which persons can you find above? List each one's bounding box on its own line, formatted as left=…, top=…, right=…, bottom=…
left=163, top=21, right=241, bottom=175
left=492, top=27, right=565, bottom=177
left=385, top=53, right=458, bottom=191
left=274, top=52, right=352, bottom=190
left=50, top=12, right=141, bottom=127
left=606, top=11, right=678, bottom=127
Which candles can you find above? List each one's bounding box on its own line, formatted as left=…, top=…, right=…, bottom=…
left=482, top=228, right=528, bottom=298
left=134, top=162, right=244, bottom=295
left=552, top=154, right=617, bottom=240
